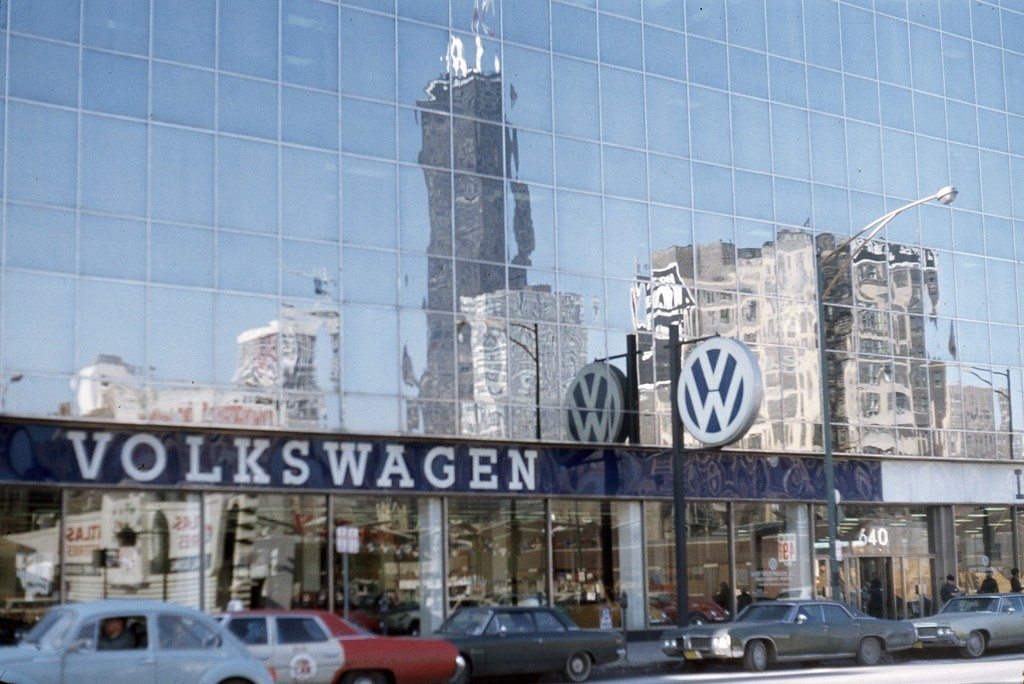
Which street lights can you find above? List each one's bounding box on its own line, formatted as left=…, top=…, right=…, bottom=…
left=814, top=185, right=960, bottom=599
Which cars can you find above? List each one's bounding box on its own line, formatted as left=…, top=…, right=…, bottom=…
left=651, top=590, right=727, bottom=625
left=426, top=606, right=627, bottom=684
left=660, top=598, right=921, bottom=673
left=207, top=608, right=465, bottom=684
left=897, top=591, right=1024, bottom=658
left=0, top=600, right=276, bottom=684
left=559, top=588, right=667, bottom=630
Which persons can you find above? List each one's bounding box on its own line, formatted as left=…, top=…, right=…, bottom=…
left=1009, top=568, right=1024, bottom=592
left=715, top=581, right=732, bottom=611
left=940, top=575, right=964, bottom=610
left=737, top=585, right=753, bottom=610
left=979, top=569, right=999, bottom=594
left=79, top=616, right=133, bottom=651
